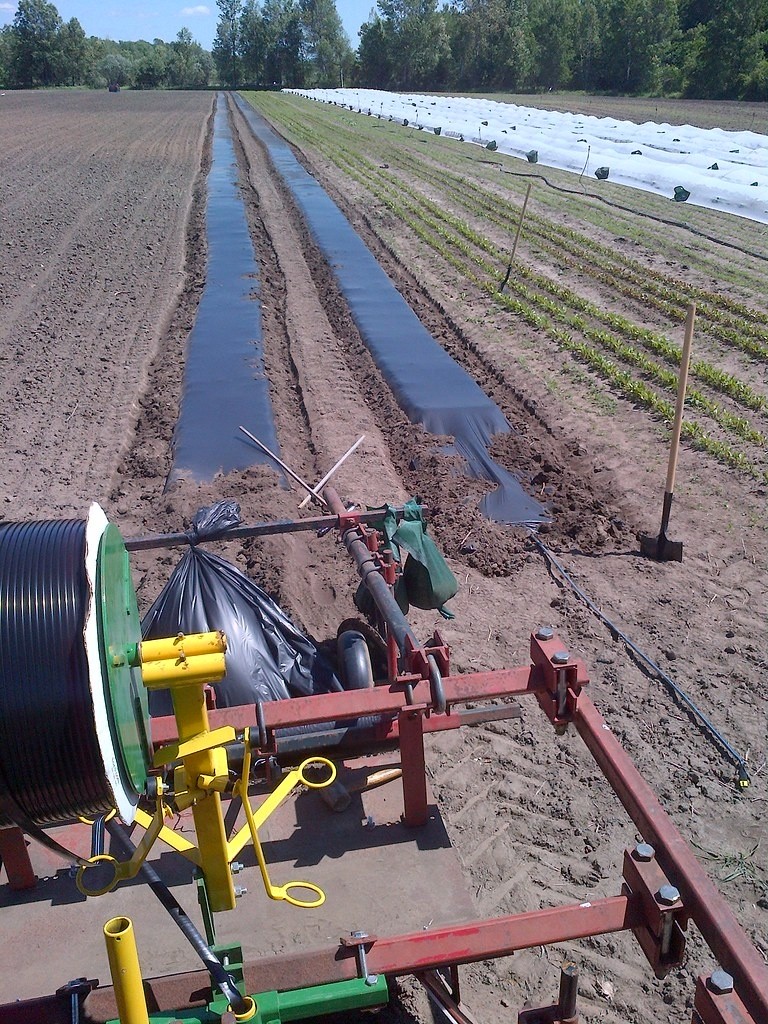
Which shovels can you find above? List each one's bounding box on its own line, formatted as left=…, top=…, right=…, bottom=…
left=498, top=183, right=533, bottom=295
left=639, top=303, right=696, bottom=563
